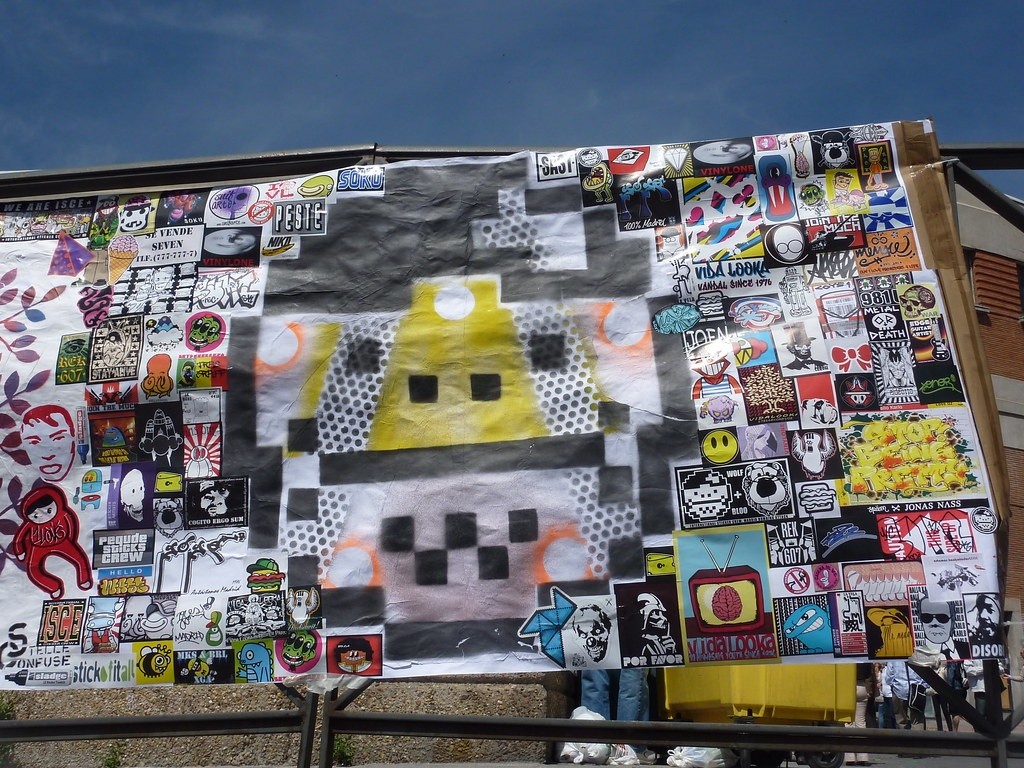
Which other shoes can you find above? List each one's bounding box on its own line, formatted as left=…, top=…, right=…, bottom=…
left=636, top=748, right=660, bottom=765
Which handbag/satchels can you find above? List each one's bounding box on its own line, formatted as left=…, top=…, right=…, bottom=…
left=666, top=746, right=739, bottom=768
left=559, top=706, right=611, bottom=765
left=908, top=683, right=926, bottom=714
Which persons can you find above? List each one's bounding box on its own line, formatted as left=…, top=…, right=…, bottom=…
left=963, top=658, right=1005, bottom=715
left=967, top=594, right=1002, bottom=644
left=865, top=660, right=970, bottom=734
left=844, top=663, right=873, bottom=766
left=579, top=666, right=660, bottom=765
left=1002, top=648, right=1024, bottom=682
